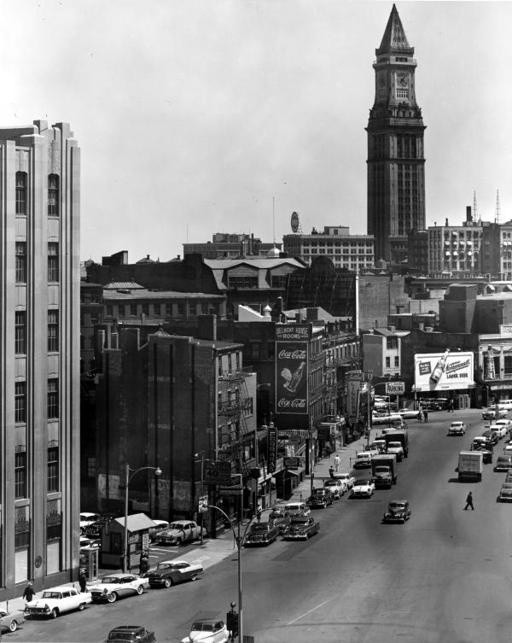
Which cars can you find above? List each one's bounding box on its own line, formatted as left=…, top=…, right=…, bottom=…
left=242, top=392, right=420, bottom=547
left=0, top=509, right=234, bottom=642
left=420, top=396, right=512, bottom=503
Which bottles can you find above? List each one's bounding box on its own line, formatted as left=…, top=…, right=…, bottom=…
left=285, top=362, right=306, bottom=393
left=431, top=348, right=452, bottom=384
left=479, top=344, right=507, bottom=381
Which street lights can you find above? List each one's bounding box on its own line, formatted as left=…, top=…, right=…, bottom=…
left=122, top=463, right=164, bottom=572
left=200, top=502, right=282, bottom=642
left=194, top=448, right=217, bottom=528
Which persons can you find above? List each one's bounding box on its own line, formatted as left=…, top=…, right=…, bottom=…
left=417, top=412, right=422, bottom=421
left=22, top=581, right=37, bottom=611
left=139, top=553, right=148, bottom=574
left=464, top=491, right=474, bottom=510
left=447, top=397, right=454, bottom=412
left=329, top=464, right=334, bottom=476
left=78, top=569, right=87, bottom=592
left=423, top=410, right=428, bottom=423
left=334, top=454, right=340, bottom=472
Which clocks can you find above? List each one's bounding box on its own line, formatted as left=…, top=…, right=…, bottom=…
left=396, top=72, right=409, bottom=88
